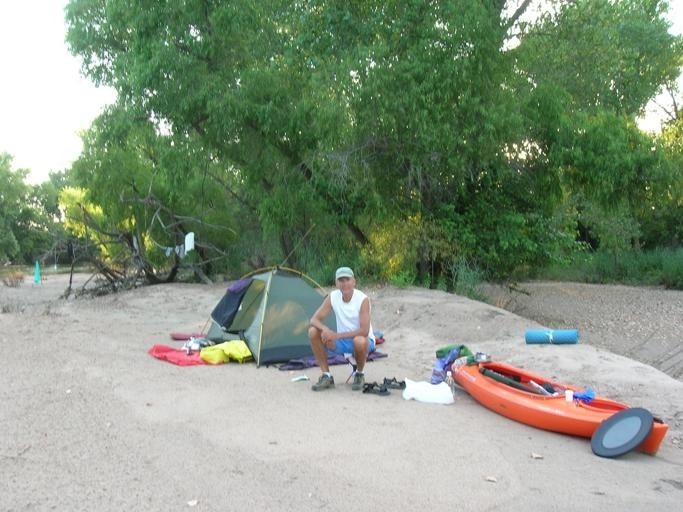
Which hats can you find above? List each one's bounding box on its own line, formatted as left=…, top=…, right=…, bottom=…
left=336, top=266, right=355, bottom=279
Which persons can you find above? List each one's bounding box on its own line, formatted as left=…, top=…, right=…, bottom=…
left=305, top=266, right=375, bottom=392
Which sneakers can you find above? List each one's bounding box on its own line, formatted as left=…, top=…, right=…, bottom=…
left=313, top=374, right=335, bottom=391
left=352, top=373, right=365, bottom=390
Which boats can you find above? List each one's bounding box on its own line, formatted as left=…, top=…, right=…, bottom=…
left=449, top=351, right=672, bottom=459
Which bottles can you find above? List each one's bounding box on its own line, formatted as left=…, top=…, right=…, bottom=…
left=444, top=370, right=455, bottom=395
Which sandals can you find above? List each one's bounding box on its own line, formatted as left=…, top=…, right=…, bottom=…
left=382, top=377, right=406, bottom=390
left=363, top=381, right=390, bottom=396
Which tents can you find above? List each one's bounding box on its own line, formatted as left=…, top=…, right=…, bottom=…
left=192, top=263, right=338, bottom=370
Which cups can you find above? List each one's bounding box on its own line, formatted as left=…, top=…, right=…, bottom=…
left=565, top=390, right=573, bottom=402
